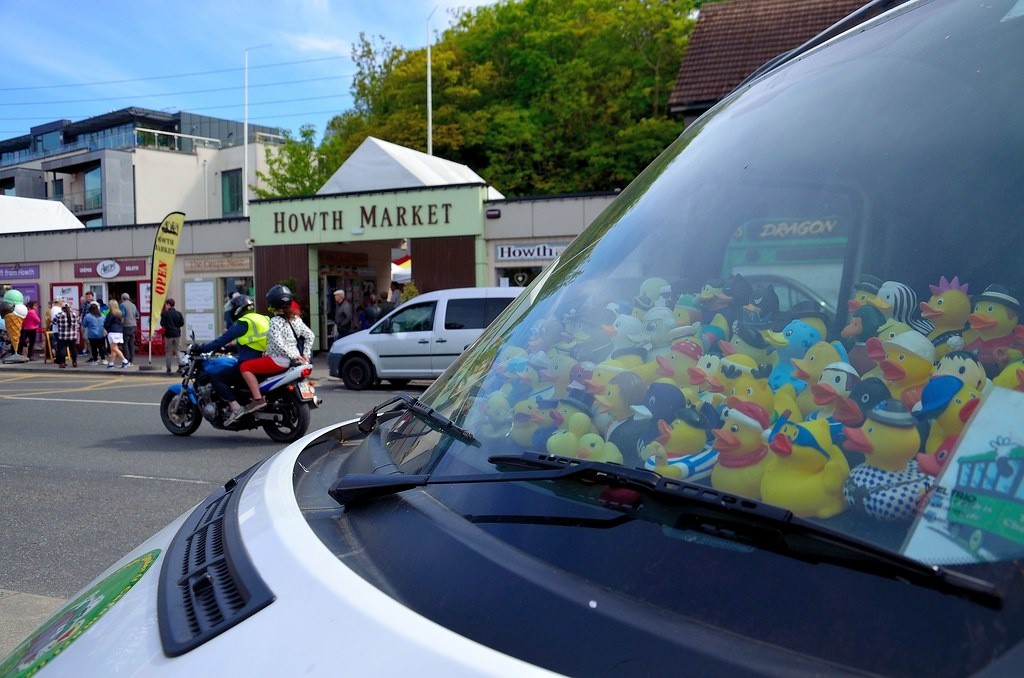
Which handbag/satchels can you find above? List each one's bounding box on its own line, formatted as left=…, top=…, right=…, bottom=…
left=296, top=335, right=314, bottom=365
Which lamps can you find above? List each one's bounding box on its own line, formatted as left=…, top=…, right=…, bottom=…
left=245, top=238, right=255, bottom=251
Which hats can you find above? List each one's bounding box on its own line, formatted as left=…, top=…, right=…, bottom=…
left=333, top=290, right=345, bottom=299
left=13, top=304, right=28, bottom=318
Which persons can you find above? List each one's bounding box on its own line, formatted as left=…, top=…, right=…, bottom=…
left=224, top=292, right=234, bottom=329
left=50, top=303, right=81, bottom=367
left=19, top=300, right=40, bottom=360
left=356, top=306, right=368, bottom=330
left=103, top=300, right=131, bottom=369
left=390, top=281, right=401, bottom=308
left=160, top=299, right=185, bottom=372
left=119, top=293, right=140, bottom=363
left=50, top=300, right=63, bottom=332
left=82, top=304, right=108, bottom=365
left=333, top=290, right=353, bottom=338
left=378, top=292, right=393, bottom=317
left=364, top=294, right=378, bottom=328
left=96, top=299, right=108, bottom=313
left=77, top=292, right=95, bottom=356
left=240, top=285, right=315, bottom=414
left=187, top=294, right=271, bottom=427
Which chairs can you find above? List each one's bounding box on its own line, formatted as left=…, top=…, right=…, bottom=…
left=135, top=329, right=166, bottom=357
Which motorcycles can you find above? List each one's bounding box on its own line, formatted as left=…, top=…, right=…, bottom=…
left=160, top=330, right=323, bottom=443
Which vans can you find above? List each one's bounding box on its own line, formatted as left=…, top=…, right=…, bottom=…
left=327, top=287, right=525, bottom=391
left=0, top=0, right=1024, bottom=678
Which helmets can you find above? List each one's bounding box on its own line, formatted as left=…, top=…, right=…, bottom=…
left=227, top=294, right=253, bottom=318
left=2, top=288, right=25, bottom=304
left=265, top=284, right=294, bottom=309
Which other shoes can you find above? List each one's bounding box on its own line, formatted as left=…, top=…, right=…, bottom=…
left=30, top=357, right=34, bottom=361
left=59, top=363, right=65, bottom=368
left=106, top=364, right=115, bottom=369
left=76, top=349, right=91, bottom=357
left=85, top=357, right=97, bottom=365
left=72, top=362, right=78, bottom=367
left=101, top=359, right=109, bottom=365
left=167, top=369, right=172, bottom=375
left=224, top=406, right=248, bottom=427
left=95, top=359, right=100, bottom=363
left=244, top=395, right=269, bottom=416
left=120, top=361, right=131, bottom=370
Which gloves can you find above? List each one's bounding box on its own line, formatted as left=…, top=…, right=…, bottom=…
left=185, top=342, right=205, bottom=356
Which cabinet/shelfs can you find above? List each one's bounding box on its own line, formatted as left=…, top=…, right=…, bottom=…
left=319, top=273, right=345, bottom=350
